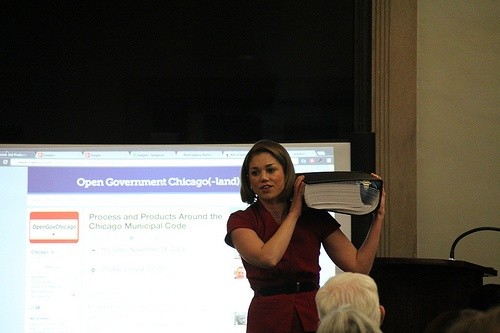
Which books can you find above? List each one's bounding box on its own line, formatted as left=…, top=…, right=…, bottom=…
left=295, top=172, right=383, bottom=216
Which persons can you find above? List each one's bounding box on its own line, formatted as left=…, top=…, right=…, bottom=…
left=224, top=140, right=386, bottom=333
left=317, top=305, right=384, bottom=333
left=316, top=273, right=385, bottom=326
